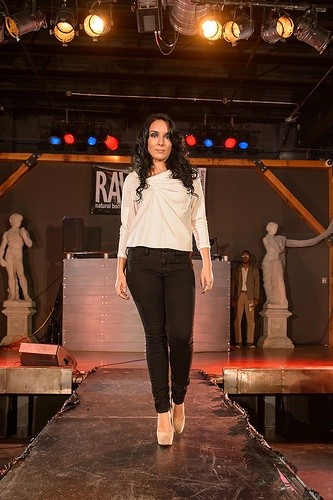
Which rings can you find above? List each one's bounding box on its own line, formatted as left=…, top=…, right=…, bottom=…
left=207, top=285, right=211, bottom=286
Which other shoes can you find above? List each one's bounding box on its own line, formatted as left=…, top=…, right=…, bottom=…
left=249, top=344, right=256, bottom=348
left=235, top=343, right=242, bottom=349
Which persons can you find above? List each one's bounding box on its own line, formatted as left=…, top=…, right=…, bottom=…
left=115, top=115, right=215, bottom=445
left=0, top=213, right=32, bottom=301
left=231, top=250, right=260, bottom=348
left=262, top=222, right=287, bottom=303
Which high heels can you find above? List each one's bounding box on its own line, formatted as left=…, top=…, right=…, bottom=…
left=174, top=404, right=185, bottom=434
left=157, top=409, right=173, bottom=445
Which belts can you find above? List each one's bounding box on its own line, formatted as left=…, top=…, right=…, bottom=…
left=241, top=291, right=247, bottom=294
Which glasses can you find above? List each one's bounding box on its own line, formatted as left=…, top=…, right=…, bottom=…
left=242, top=255, right=248, bottom=257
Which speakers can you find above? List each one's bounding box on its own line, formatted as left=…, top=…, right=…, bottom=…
left=19, top=343, right=78, bottom=370
left=62, top=217, right=83, bottom=251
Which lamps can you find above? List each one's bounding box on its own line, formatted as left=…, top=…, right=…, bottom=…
left=183, top=124, right=251, bottom=150
left=0, top=0, right=333, bottom=55
left=48, top=126, right=121, bottom=152
